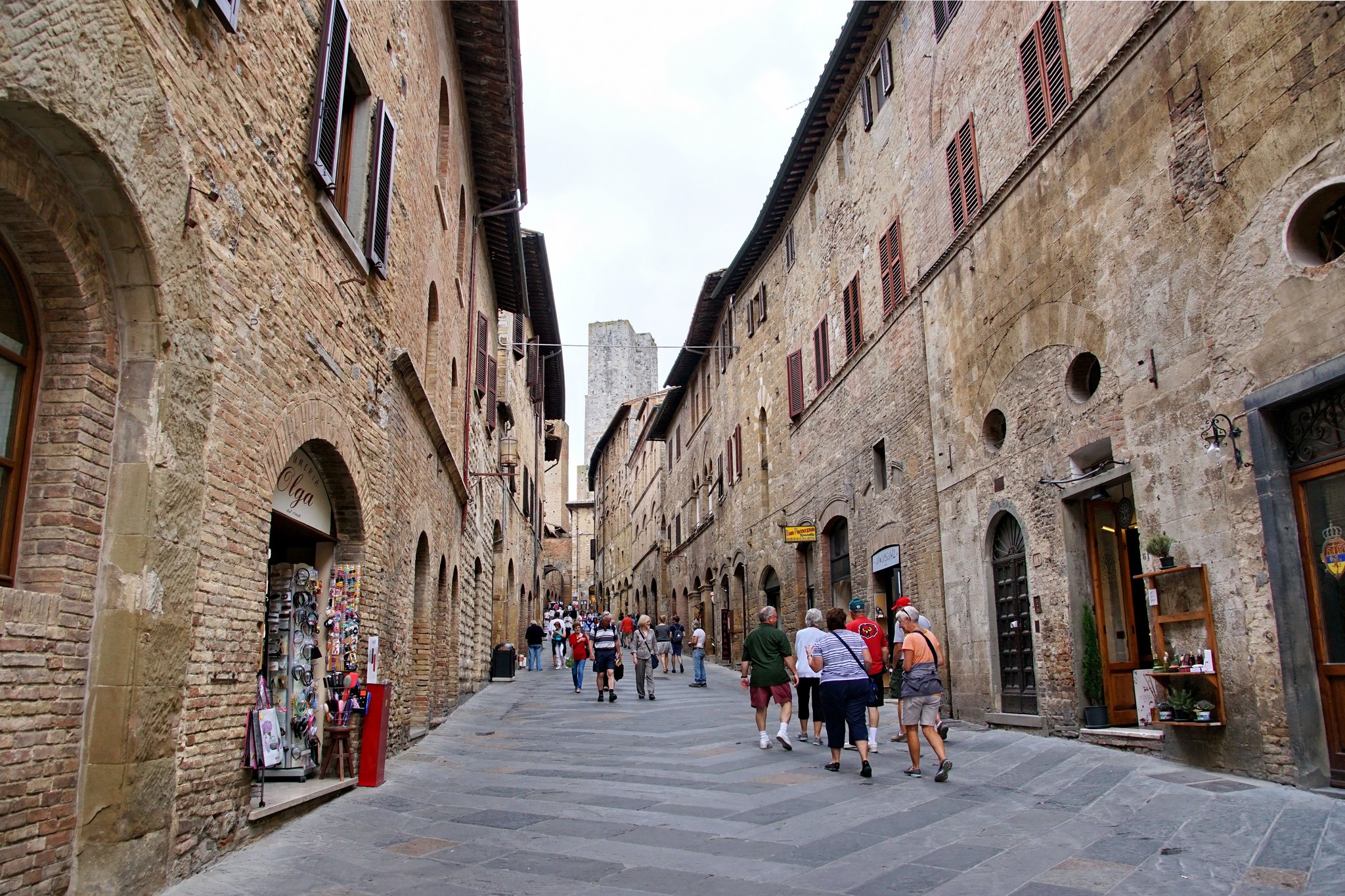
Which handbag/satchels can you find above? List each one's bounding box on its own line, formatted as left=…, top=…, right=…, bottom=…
left=614, top=658, right=624, bottom=682
left=583, top=634, right=590, bottom=659
left=565, top=645, right=575, bottom=668
left=867, top=678, right=879, bottom=703
left=625, top=598, right=628, bottom=606
left=243, top=674, right=283, bottom=768
left=651, top=654, right=659, bottom=669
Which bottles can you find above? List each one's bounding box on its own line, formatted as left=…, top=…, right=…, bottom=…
left=1164, top=653, right=1171, bottom=667
left=1197, top=649, right=1203, bottom=665
left=1163, top=656, right=1169, bottom=673
left=1187, top=651, right=1195, bottom=668
left=1153, top=654, right=1160, bottom=667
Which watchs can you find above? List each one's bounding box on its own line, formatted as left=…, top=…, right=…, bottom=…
left=741, top=675, right=748, bottom=679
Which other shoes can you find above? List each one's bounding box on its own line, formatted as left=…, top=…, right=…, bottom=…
left=890, top=731, right=907, bottom=742
left=903, top=759, right=952, bottom=777
left=796, top=730, right=823, bottom=745
left=573, top=683, right=656, bottom=701
left=936, top=722, right=949, bottom=740
left=824, top=762, right=872, bottom=778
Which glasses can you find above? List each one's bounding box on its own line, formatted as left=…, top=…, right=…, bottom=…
left=631, top=615, right=634, bottom=616
left=895, top=606, right=901, bottom=611
left=602, top=613, right=611, bottom=619
left=838, top=608, right=843, bottom=615
left=900, top=607, right=911, bottom=621
left=767, top=615, right=778, bottom=620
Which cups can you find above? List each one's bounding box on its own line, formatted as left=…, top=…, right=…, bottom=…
left=688, top=642, right=693, bottom=649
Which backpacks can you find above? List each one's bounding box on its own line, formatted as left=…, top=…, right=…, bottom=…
left=672, top=624, right=684, bottom=642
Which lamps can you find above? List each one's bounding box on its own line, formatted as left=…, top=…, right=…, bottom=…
left=1200, top=414, right=1242, bottom=469
left=468, top=436, right=520, bottom=505
left=562, top=568, right=572, bottom=576
left=554, top=579, right=562, bottom=585
left=662, top=539, right=687, bottom=573
left=542, top=555, right=553, bottom=569
left=1091, top=490, right=1111, bottom=500
left=586, top=566, right=598, bottom=580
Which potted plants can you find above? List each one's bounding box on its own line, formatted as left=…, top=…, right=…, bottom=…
left=1166, top=685, right=1197, bottom=722
left=1082, top=601, right=1112, bottom=729
left=1156, top=701, right=1175, bottom=722
left=1192, top=699, right=1215, bottom=720
left=1145, top=532, right=1177, bottom=569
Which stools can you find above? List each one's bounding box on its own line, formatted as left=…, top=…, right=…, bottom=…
left=319, top=725, right=356, bottom=781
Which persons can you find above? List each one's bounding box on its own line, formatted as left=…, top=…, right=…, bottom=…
left=630, top=614, right=660, bottom=700
left=588, top=611, right=622, bottom=702
left=889, top=596, right=948, bottom=742
left=569, top=622, right=589, bottom=692
left=552, top=621, right=566, bottom=670
left=805, top=607, right=872, bottom=777
left=525, top=620, right=547, bottom=671
left=740, top=606, right=799, bottom=750
left=688, top=621, right=708, bottom=687
left=543, top=601, right=640, bottom=649
left=895, top=605, right=951, bottom=782
left=670, top=615, right=685, bottom=673
left=795, top=608, right=828, bottom=745
left=655, top=615, right=673, bottom=673
left=843, top=597, right=889, bottom=753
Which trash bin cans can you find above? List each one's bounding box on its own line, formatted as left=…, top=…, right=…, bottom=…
left=490, top=643, right=517, bottom=682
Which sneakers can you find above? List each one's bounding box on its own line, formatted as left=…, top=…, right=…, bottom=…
left=689, top=682, right=707, bottom=687
left=844, top=738, right=878, bottom=751
left=528, top=663, right=568, bottom=672
left=760, top=730, right=793, bottom=751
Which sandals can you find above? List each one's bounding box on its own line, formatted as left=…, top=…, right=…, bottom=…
left=663, top=663, right=685, bottom=673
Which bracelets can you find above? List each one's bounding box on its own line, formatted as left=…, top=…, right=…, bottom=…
left=807, top=655, right=812, bottom=659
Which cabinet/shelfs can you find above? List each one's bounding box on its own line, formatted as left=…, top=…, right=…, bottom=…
left=1132, top=562, right=1227, bottom=726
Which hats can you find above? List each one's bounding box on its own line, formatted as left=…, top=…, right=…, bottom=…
left=849, top=598, right=865, bottom=610
left=891, top=597, right=912, bottom=610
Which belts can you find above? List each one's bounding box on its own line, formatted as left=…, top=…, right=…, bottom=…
left=693, top=647, right=703, bottom=650
